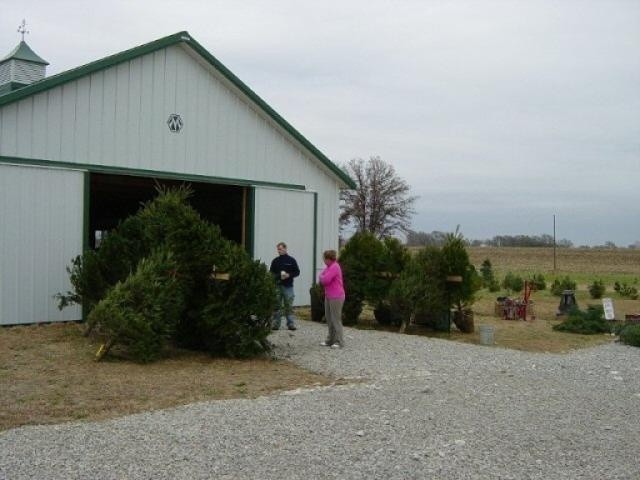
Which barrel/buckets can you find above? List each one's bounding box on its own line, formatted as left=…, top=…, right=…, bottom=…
left=478, top=325, right=494, bottom=345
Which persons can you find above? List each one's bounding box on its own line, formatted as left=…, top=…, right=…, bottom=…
left=318, top=248, right=347, bottom=349
left=267, top=242, right=301, bottom=332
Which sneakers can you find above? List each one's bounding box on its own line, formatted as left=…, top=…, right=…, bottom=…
left=271, top=321, right=296, bottom=330
left=320, top=339, right=344, bottom=348
